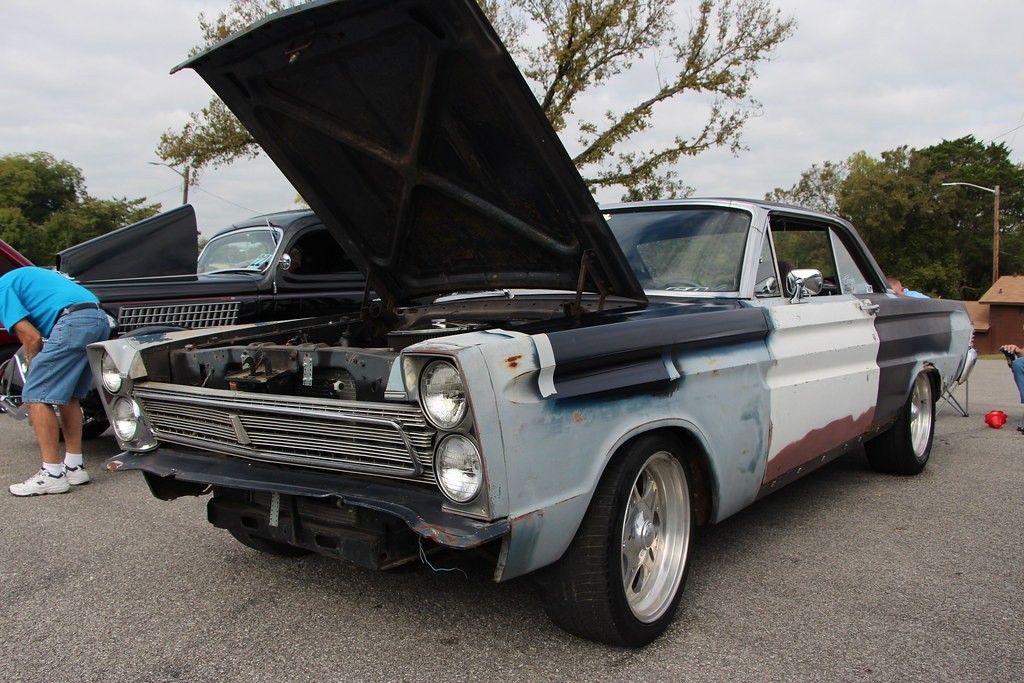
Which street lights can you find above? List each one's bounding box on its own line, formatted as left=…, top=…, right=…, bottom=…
left=941, top=181, right=1000, bottom=286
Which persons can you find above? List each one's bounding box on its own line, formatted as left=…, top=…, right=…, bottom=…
left=0, top=266, right=110, bottom=496
left=887, top=277, right=930, bottom=298
left=1000, top=345, right=1024, bottom=435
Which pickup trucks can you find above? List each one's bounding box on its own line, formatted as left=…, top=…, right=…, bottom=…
left=73, top=207, right=360, bottom=426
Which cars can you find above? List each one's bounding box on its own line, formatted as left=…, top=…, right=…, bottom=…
left=1, top=241, right=50, bottom=413
left=82, top=0, right=978, bottom=651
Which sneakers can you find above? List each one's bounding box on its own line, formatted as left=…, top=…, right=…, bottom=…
left=63, top=463, right=90, bottom=486
left=8, top=466, right=70, bottom=497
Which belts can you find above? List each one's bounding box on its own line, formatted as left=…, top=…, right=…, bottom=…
left=55, top=303, right=103, bottom=324
